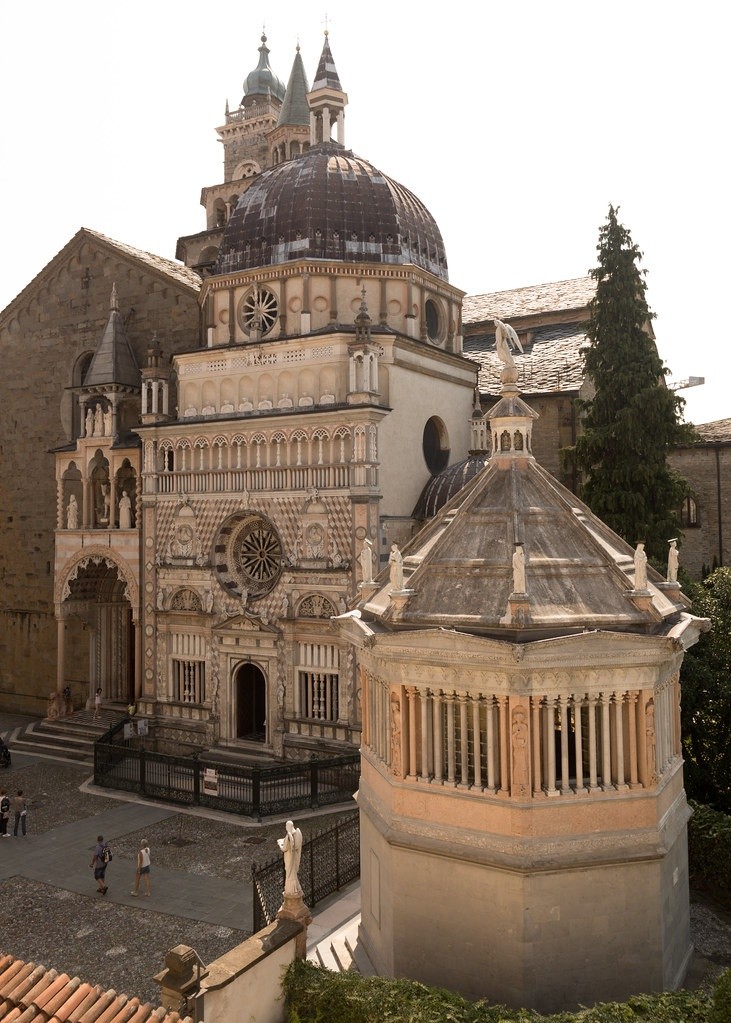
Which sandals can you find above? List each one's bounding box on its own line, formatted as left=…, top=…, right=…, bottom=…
left=102, top=886, right=108, bottom=895
left=97, top=888, right=104, bottom=892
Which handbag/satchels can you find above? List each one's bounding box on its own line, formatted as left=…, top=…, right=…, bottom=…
left=20, top=810, right=27, bottom=816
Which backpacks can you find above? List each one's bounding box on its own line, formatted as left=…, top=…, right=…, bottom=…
left=97, top=844, right=112, bottom=863
left=1, top=797, right=10, bottom=812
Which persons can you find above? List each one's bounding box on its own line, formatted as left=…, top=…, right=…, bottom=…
left=361, top=540, right=373, bottom=584
left=67, top=403, right=131, bottom=529
left=645, top=705, right=657, bottom=775
left=492, top=320, right=513, bottom=367
left=87, top=836, right=109, bottom=895
left=388, top=545, right=404, bottom=590
left=311, top=485, right=318, bottom=503
left=64, top=686, right=136, bottom=721
left=180, top=491, right=188, bottom=506
left=634, top=544, right=649, bottom=590
left=391, top=701, right=400, bottom=774
left=130, top=839, right=151, bottom=897
left=513, top=546, right=526, bottom=593
left=0, top=789, right=28, bottom=838
left=512, top=713, right=528, bottom=784
left=243, top=489, right=250, bottom=509
left=667, top=542, right=680, bottom=581
left=279, top=820, right=302, bottom=895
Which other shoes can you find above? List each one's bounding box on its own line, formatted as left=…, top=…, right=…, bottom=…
left=24, top=833, right=27, bottom=837
left=0, top=833, right=3, bottom=835
left=14, top=836, right=17, bottom=838
left=145, top=892, right=150, bottom=896
left=2, top=833, right=11, bottom=837
left=131, top=891, right=138, bottom=896
